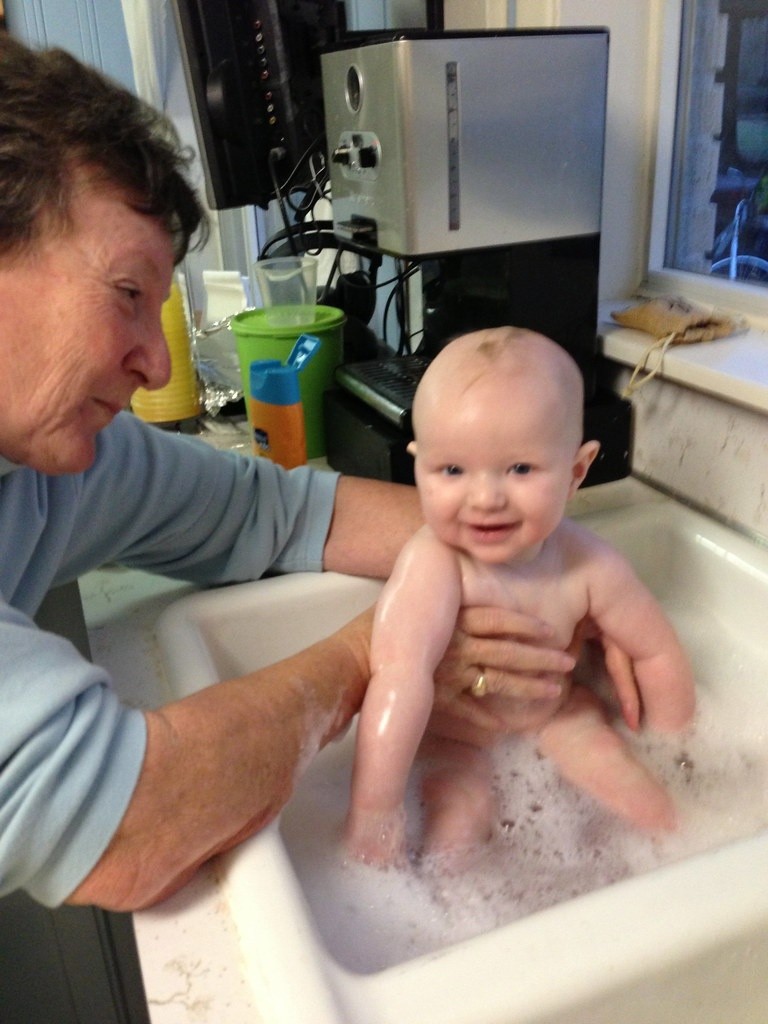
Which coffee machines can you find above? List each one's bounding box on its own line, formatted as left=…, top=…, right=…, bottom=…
left=324, top=25, right=635, bottom=488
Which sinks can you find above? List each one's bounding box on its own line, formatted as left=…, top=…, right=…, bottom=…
left=152, top=471, right=768, bottom=1024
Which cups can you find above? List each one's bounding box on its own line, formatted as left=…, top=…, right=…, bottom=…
left=232, top=308, right=347, bottom=459
left=255, top=257, right=319, bottom=326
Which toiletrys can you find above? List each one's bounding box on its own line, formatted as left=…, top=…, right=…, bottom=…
left=248, top=332, right=325, bottom=469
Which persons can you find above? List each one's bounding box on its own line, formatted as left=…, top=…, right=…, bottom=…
left=0, top=27, right=640, bottom=915
left=346, top=325, right=696, bottom=817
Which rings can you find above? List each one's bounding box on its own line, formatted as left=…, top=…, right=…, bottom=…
left=471, top=666, right=487, bottom=698
